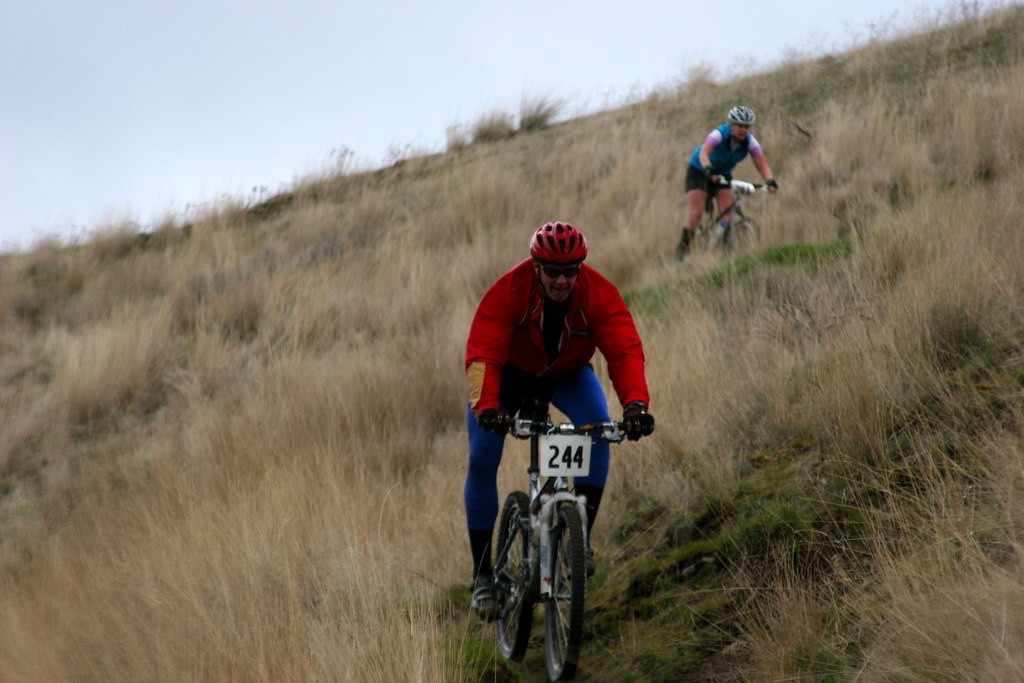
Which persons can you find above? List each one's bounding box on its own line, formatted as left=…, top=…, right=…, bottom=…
left=673, top=105, right=780, bottom=261
left=461, top=219, right=656, bottom=621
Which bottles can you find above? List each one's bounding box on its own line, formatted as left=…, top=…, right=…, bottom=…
left=712, top=222, right=727, bottom=244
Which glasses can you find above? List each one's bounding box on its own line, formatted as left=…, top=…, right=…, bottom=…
left=731, top=122, right=751, bottom=129
left=538, top=261, right=581, bottom=279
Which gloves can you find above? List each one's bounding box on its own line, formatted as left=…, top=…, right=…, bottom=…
left=622, top=400, right=656, bottom=442
left=477, top=408, right=517, bottom=436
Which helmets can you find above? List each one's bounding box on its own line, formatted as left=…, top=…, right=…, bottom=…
left=529, top=221, right=589, bottom=263
left=728, top=107, right=757, bottom=126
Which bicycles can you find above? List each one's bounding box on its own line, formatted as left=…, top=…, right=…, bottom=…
left=678, top=173, right=776, bottom=251
left=466, top=399, right=641, bottom=683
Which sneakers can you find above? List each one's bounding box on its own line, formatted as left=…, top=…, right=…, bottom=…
left=470, top=574, right=500, bottom=621
left=564, top=538, right=594, bottom=579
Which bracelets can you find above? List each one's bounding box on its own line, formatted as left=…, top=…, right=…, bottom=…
left=623, top=406, right=646, bottom=417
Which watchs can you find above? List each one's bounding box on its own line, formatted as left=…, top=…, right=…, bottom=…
left=625, top=400, right=647, bottom=409
